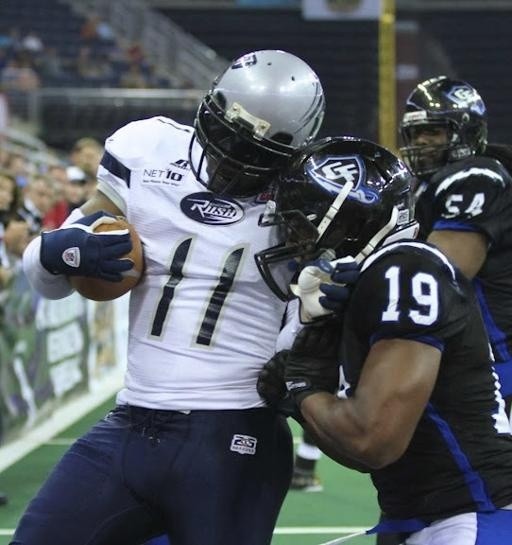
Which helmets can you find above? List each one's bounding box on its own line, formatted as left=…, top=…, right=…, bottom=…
left=274, top=136, right=413, bottom=275
left=196, top=47, right=326, bottom=199
left=399, top=75, right=489, bottom=165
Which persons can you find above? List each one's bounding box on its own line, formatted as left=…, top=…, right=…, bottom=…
left=0, top=137, right=104, bottom=289
left=255, top=135, right=510, bottom=545
left=389, top=72, right=511, bottom=356
left=7, top=47, right=326, bottom=543
left=0, top=13, right=179, bottom=97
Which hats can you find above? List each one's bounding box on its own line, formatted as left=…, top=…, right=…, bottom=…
left=65, top=164, right=85, bottom=183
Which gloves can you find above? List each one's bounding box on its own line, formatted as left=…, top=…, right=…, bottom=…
left=37, top=209, right=136, bottom=286
left=287, top=255, right=360, bottom=324
left=254, top=350, right=340, bottom=413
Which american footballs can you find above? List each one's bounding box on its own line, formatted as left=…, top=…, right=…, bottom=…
left=66, top=216, right=143, bottom=301
